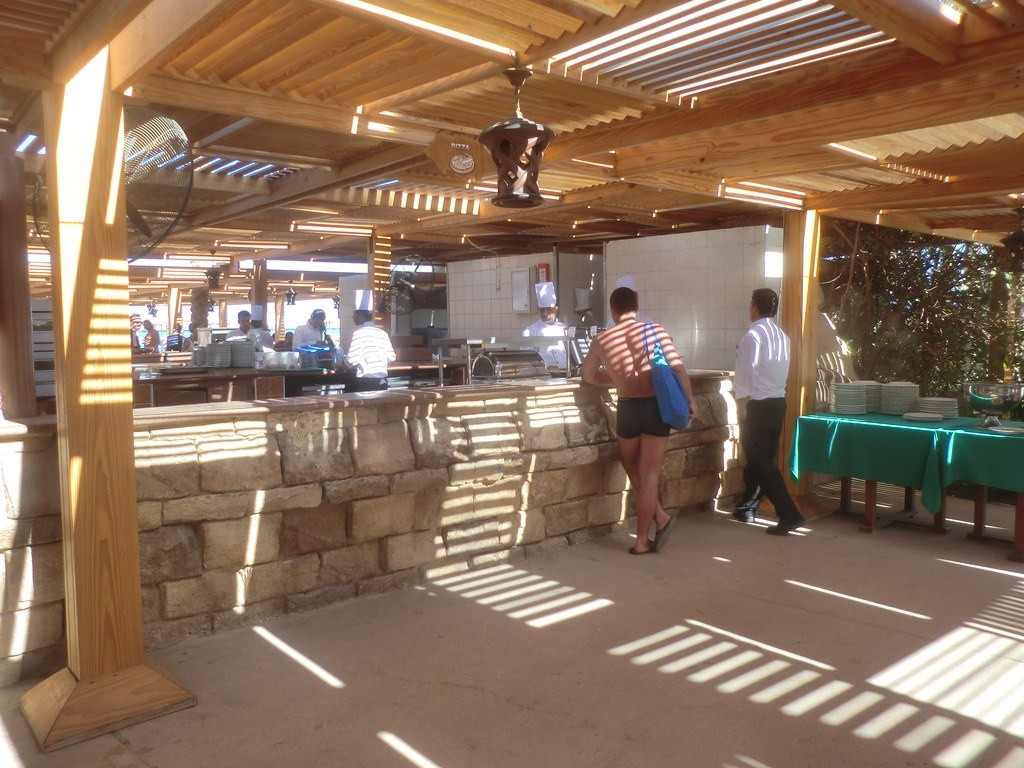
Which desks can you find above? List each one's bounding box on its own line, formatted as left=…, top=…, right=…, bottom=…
left=787, top=411, right=1023, bottom=564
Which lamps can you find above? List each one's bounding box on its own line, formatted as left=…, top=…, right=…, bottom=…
left=479, top=69, right=550, bottom=207
left=1000, top=206, right=1024, bottom=261
left=128, top=222, right=376, bottom=312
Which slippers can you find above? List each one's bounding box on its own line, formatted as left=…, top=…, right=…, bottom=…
left=630, top=541, right=656, bottom=556
left=653, top=516, right=676, bottom=551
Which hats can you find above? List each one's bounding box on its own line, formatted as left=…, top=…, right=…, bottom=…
left=354, top=289, right=374, bottom=312
left=535, top=282, right=556, bottom=308
left=251, top=304, right=264, bottom=321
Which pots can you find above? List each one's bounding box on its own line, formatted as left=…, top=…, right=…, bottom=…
left=955, top=382, right=1024, bottom=429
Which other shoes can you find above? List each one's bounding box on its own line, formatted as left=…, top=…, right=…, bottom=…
left=767, top=516, right=805, bottom=536
left=732, top=506, right=756, bottom=523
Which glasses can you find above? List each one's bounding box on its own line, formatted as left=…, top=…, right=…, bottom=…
left=132, top=321, right=142, bottom=324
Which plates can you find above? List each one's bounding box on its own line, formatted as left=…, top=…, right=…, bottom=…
left=987, top=426, right=1023, bottom=434
left=191, top=339, right=256, bottom=368
left=254, top=349, right=299, bottom=369
left=915, top=396, right=959, bottom=419
left=828, top=383, right=866, bottom=415
left=880, top=381, right=920, bottom=415
left=853, top=381, right=881, bottom=413
left=902, top=412, right=944, bottom=421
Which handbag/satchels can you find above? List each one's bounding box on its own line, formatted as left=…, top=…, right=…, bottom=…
left=643, top=322, right=690, bottom=432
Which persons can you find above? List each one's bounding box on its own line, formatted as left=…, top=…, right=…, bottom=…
left=183, top=322, right=199, bottom=353
left=731, top=288, right=806, bottom=536
left=291, top=309, right=326, bottom=353
left=161, top=323, right=187, bottom=353
left=520, top=282, right=578, bottom=379
left=142, top=319, right=161, bottom=354
left=130, top=313, right=142, bottom=355
left=343, top=290, right=397, bottom=393
left=579, top=286, right=695, bottom=555
left=224, top=304, right=294, bottom=355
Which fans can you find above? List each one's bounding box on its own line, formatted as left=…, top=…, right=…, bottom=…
left=31, top=104, right=195, bottom=261
left=378, top=252, right=437, bottom=315
